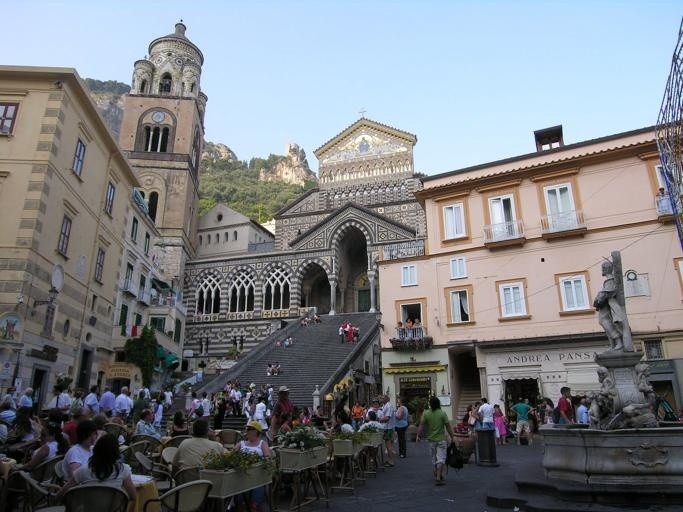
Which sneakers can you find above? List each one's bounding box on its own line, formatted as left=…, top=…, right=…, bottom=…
left=434, top=472, right=447, bottom=485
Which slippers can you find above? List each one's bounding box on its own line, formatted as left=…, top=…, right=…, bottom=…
left=384, top=461, right=394, bottom=467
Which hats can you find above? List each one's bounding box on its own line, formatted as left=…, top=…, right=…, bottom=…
left=279, top=386, right=290, bottom=392
left=244, top=421, right=262, bottom=433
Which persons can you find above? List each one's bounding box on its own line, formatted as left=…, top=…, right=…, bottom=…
left=277, top=336, right=294, bottom=349
left=301, top=314, right=321, bottom=327
left=265, top=361, right=281, bottom=376
left=593, top=261, right=625, bottom=351
left=655, top=187, right=669, bottom=214
left=338, top=321, right=361, bottom=345
left=0, top=380, right=683, bottom=512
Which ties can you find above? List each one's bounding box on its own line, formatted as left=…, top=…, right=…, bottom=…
left=56, top=395, right=59, bottom=407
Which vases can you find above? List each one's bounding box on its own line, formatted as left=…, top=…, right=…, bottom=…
left=448, top=433, right=475, bottom=463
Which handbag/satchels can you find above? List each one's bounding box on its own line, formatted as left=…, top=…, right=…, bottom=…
left=467, top=416, right=476, bottom=425
left=552, top=407, right=560, bottom=424
left=447, top=441, right=463, bottom=468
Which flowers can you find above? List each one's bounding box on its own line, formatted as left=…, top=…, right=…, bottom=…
left=453, top=423, right=469, bottom=433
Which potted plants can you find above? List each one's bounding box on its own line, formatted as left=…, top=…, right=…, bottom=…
left=331, top=431, right=365, bottom=456
left=360, top=423, right=385, bottom=447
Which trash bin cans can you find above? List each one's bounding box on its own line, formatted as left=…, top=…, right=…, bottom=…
left=475, top=428, right=500, bottom=467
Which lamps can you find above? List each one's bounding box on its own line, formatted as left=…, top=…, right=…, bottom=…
left=34, top=286, right=59, bottom=308
